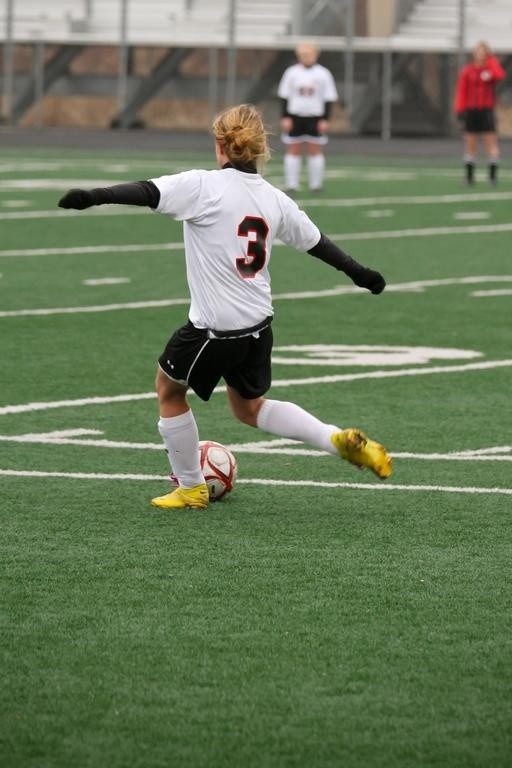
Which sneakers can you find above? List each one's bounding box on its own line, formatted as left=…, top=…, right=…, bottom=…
left=331, top=427, right=392, bottom=479
left=150, top=484, right=209, bottom=509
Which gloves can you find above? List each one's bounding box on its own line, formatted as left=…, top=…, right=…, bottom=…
left=57, top=189, right=93, bottom=209
left=336, top=254, right=386, bottom=294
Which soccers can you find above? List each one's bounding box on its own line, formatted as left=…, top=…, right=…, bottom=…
left=197, top=440, right=238, bottom=502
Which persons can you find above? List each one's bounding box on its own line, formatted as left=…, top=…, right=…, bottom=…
left=56, top=104, right=393, bottom=510
left=455, top=40, right=506, bottom=185
left=277, top=40, right=339, bottom=195
